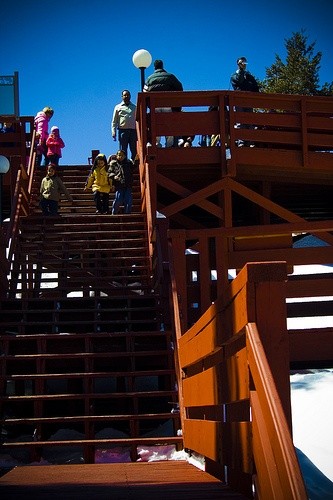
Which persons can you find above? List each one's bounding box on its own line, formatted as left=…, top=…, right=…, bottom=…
left=174, top=101, right=277, bottom=148
left=142, top=60, right=182, bottom=147
left=177, top=136, right=194, bottom=147
left=82, top=154, right=113, bottom=215
left=44, top=126, right=64, bottom=167
left=33, top=106, right=54, bottom=164
left=0, top=123, right=15, bottom=147
left=110, top=90, right=136, bottom=162
left=231, top=57, right=259, bottom=146
left=106, top=150, right=132, bottom=215
left=39, top=163, right=72, bottom=218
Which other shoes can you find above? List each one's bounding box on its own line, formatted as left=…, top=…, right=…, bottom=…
left=103, top=211, right=108, bottom=214
left=96, top=210, right=102, bottom=215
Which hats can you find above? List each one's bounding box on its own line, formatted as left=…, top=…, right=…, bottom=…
left=43, top=106, right=54, bottom=114
left=46, top=164, right=56, bottom=170
left=51, top=126, right=59, bottom=132
